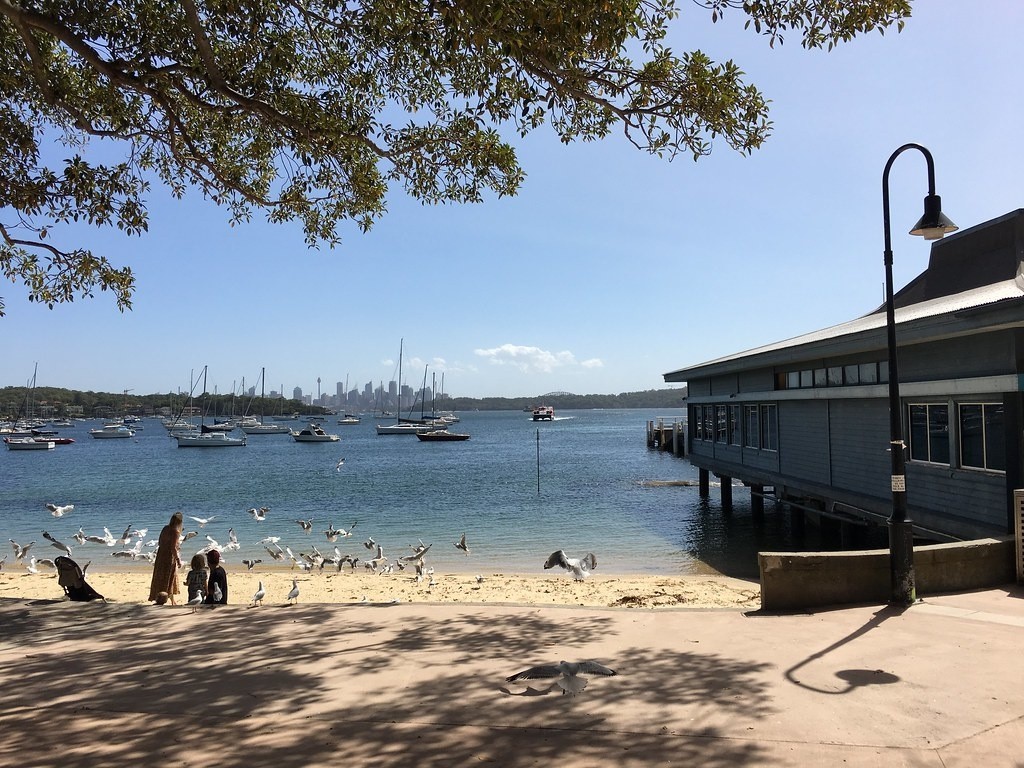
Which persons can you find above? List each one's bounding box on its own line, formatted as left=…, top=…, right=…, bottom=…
left=205, top=549, right=228, bottom=604
left=147, top=512, right=183, bottom=606
left=184, top=554, right=208, bottom=604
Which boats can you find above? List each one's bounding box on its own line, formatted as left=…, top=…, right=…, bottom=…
left=0, top=362, right=76, bottom=450
left=86, top=414, right=144, bottom=439
left=288, top=423, right=341, bottom=442
left=337, top=373, right=364, bottom=425
left=523, top=401, right=554, bottom=421
left=300, top=396, right=328, bottom=423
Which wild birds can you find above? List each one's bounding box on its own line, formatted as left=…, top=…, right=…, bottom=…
left=335, top=457, right=347, bottom=472
left=505, top=660, right=618, bottom=698
left=0, top=502, right=598, bottom=613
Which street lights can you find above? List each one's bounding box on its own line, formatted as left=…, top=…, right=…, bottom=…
left=881, top=143, right=960, bottom=605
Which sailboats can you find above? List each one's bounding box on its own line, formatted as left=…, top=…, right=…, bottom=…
left=370, top=381, right=397, bottom=419
left=161, top=365, right=291, bottom=448
left=375, top=337, right=470, bottom=440
left=271, top=384, right=297, bottom=420
